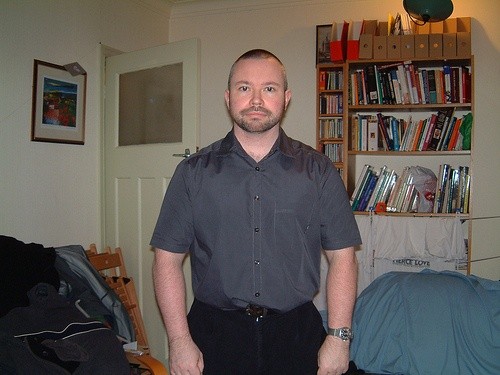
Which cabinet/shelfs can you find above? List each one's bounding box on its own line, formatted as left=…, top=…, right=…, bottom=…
left=452, top=220, right=475, bottom=275
left=314, top=18, right=476, bottom=219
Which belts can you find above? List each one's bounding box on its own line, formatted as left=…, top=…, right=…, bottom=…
left=228, top=306, right=278, bottom=319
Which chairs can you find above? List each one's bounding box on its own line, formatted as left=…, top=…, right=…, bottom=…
left=81, top=241, right=166, bottom=374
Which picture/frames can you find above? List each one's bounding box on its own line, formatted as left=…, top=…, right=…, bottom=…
left=30, top=58, right=87, bottom=146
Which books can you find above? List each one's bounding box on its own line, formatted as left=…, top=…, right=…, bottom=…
left=350, top=108, right=473, bottom=150
left=318, top=93, right=343, bottom=115
left=347, top=60, right=471, bottom=105
left=319, top=69, right=343, bottom=90
left=320, top=143, right=344, bottom=163
left=349, top=164, right=471, bottom=214
left=318, top=119, right=343, bottom=138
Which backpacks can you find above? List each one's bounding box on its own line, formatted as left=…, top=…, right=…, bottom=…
left=1, top=283, right=132, bottom=375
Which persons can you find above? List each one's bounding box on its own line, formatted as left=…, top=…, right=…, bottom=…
left=149, top=48, right=362, bottom=375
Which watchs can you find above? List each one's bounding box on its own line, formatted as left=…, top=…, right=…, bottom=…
left=327, top=327, right=354, bottom=340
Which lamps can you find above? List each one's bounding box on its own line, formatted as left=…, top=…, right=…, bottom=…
left=403, top=0, right=454, bottom=22
